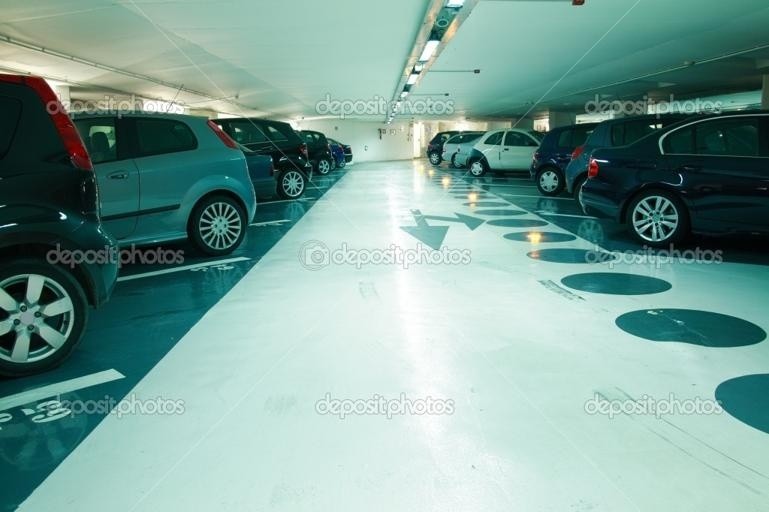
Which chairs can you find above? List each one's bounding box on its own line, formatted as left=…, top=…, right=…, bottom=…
left=88, top=131, right=111, bottom=163
